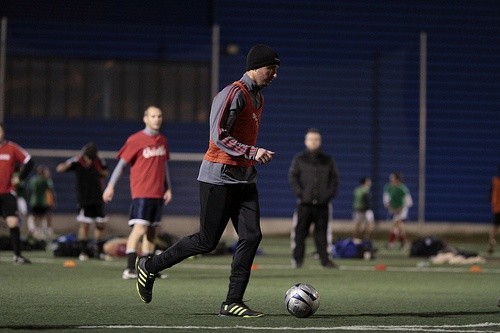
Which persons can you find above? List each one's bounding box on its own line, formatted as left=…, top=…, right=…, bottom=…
left=382, top=172, right=412, bottom=252
left=486, top=173, right=500, bottom=256
left=56, top=143, right=113, bottom=262
left=350, top=176, right=376, bottom=246
left=0, top=122, right=54, bottom=264
left=102, top=106, right=173, bottom=280
left=287, top=128, right=342, bottom=272
left=134, top=42, right=282, bottom=317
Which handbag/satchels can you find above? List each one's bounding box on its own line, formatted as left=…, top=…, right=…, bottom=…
left=0, top=235, right=45, bottom=252
left=102, top=236, right=131, bottom=258
left=331, top=235, right=373, bottom=260
left=52, top=234, right=82, bottom=257
left=410, top=237, right=439, bottom=257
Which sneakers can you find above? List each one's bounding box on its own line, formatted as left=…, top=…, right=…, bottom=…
left=486, top=239, right=497, bottom=254
left=14, top=255, right=32, bottom=264
left=98, top=253, right=113, bottom=261
left=122, top=268, right=138, bottom=279
left=79, top=252, right=88, bottom=261
left=291, top=260, right=303, bottom=271
left=134, top=256, right=156, bottom=304
left=384, top=240, right=409, bottom=252
left=218, top=302, right=265, bottom=318
left=320, top=262, right=338, bottom=269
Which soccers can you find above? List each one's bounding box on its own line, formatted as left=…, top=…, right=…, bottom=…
left=284, top=283, right=319, bottom=318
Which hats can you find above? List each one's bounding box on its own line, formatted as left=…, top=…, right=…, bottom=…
left=247, top=43, right=281, bottom=70
